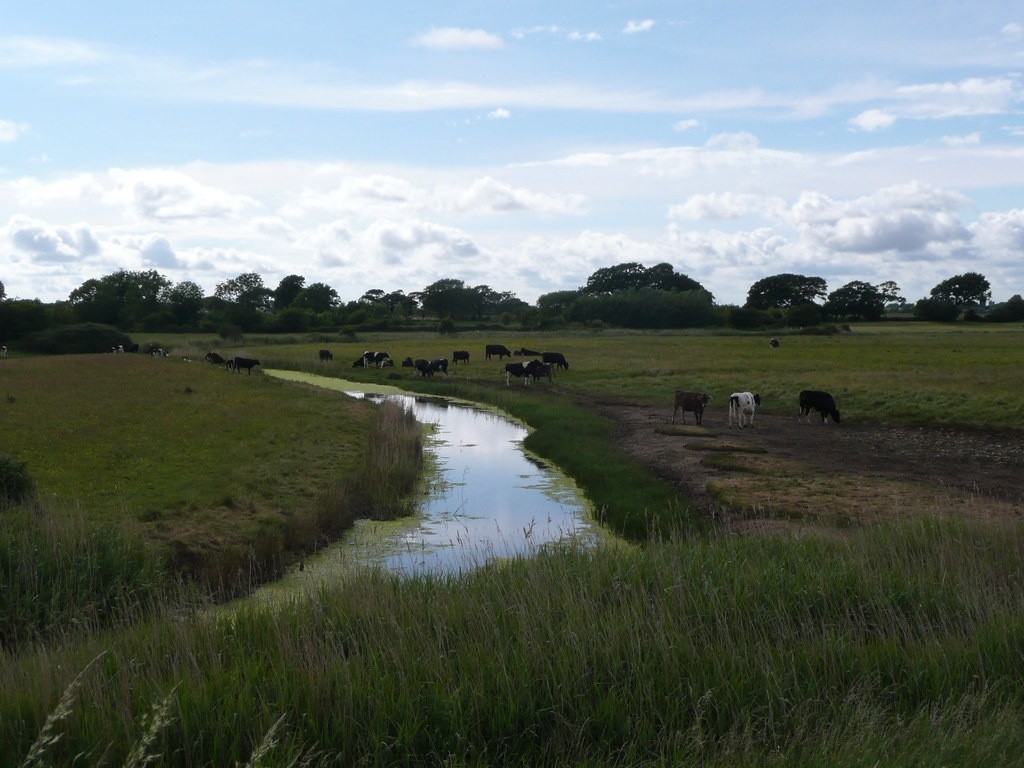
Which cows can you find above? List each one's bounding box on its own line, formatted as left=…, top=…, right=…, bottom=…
left=205, top=352, right=261, bottom=375
left=319, top=349, right=334, bottom=363
left=769, top=338, right=779, bottom=348
left=485, top=343, right=569, bottom=387
left=352, top=350, right=470, bottom=380
left=151, top=347, right=171, bottom=359
left=111, top=344, right=128, bottom=355
left=799, top=389, right=842, bottom=427
left=670, top=390, right=714, bottom=426
left=726, top=392, right=764, bottom=428
